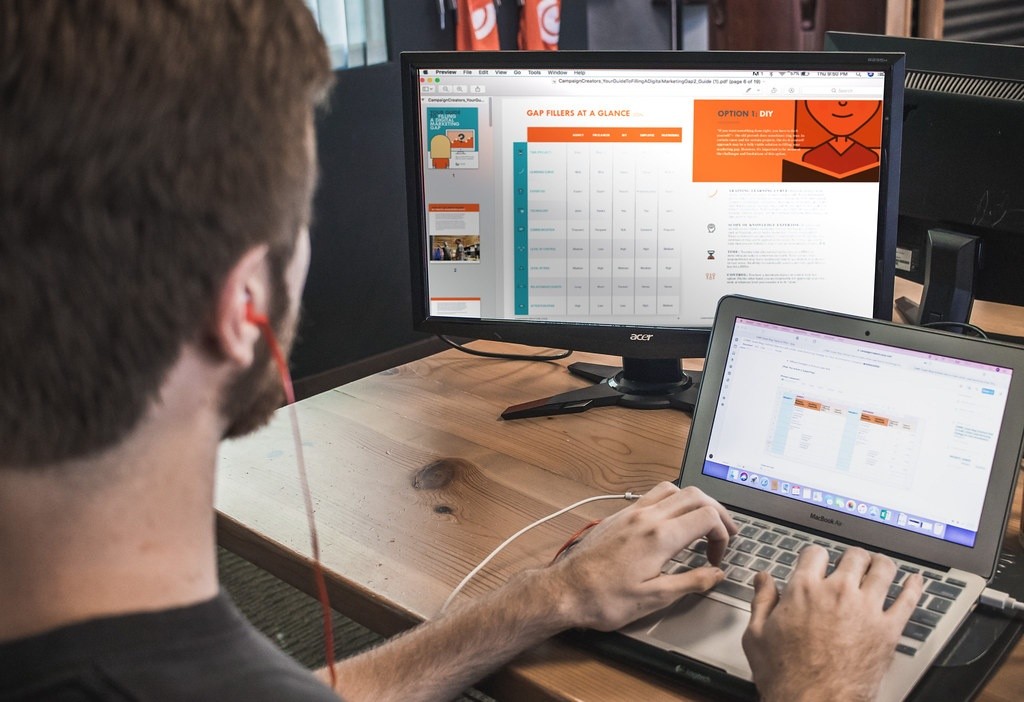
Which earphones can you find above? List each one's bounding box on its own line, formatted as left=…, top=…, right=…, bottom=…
left=244, top=296, right=271, bottom=328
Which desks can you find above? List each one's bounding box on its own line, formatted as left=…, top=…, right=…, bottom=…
left=213, top=277, right=1024, bottom=702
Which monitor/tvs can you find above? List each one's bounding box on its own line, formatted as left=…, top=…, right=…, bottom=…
left=401, top=49, right=907, bottom=419
left=821, top=29, right=1024, bottom=345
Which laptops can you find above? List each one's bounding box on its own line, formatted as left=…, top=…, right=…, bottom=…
left=576, top=293, right=1023, bottom=701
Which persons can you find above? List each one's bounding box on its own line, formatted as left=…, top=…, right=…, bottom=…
left=1, top=0, right=928, bottom=702
